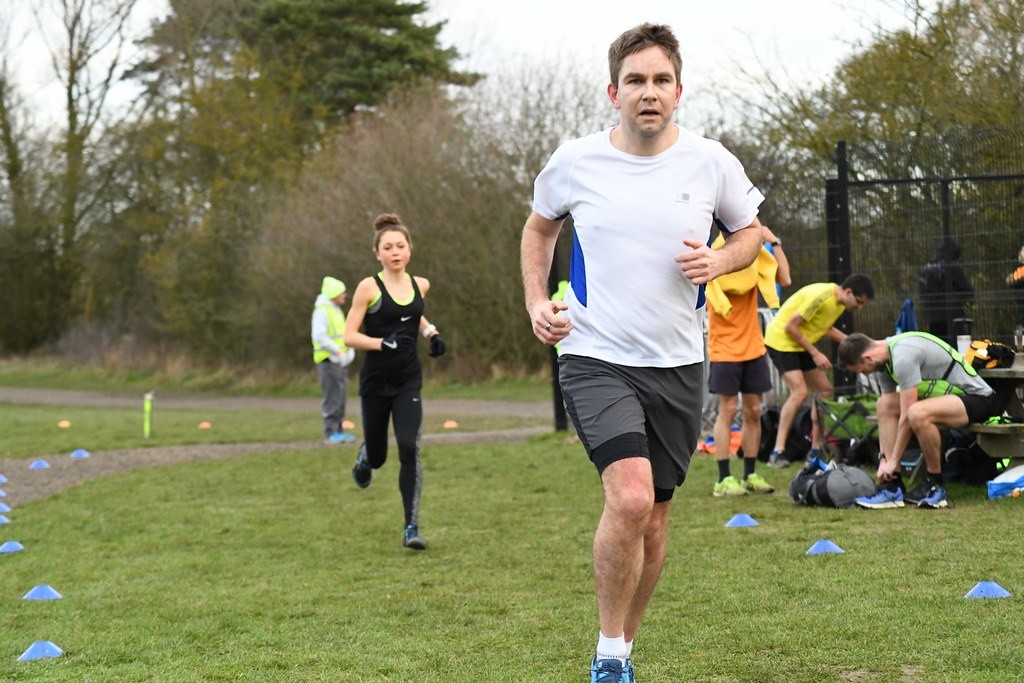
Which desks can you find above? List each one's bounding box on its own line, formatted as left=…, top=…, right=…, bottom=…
left=978, top=365, right=1024, bottom=422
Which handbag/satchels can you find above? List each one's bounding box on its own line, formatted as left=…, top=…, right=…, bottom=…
left=737, top=406, right=812, bottom=462
left=699, top=424, right=741, bottom=455
left=943, top=337, right=1024, bottom=499
left=789, top=463, right=876, bottom=508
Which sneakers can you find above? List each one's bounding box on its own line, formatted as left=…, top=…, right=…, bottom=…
left=854, top=483, right=905, bottom=509
left=588, top=653, right=636, bottom=683
left=805, top=451, right=819, bottom=465
left=917, top=474, right=948, bottom=508
left=767, top=452, right=789, bottom=468
left=741, top=473, right=776, bottom=493
left=403, top=522, right=426, bottom=548
left=353, top=441, right=373, bottom=488
left=713, top=475, right=750, bottom=497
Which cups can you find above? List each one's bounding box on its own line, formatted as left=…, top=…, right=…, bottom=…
left=957, top=335, right=971, bottom=357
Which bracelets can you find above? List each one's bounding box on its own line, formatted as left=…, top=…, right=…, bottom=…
left=878, top=452, right=885, bottom=460
left=423, top=323, right=436, bottom=338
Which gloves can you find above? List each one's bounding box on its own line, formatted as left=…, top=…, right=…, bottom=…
left=429, top=334, right=446, bottom=357
left=382, top=328, right=415, bottom=352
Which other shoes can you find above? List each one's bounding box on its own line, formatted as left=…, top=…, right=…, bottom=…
left=322, top=431, right=355, bottom=444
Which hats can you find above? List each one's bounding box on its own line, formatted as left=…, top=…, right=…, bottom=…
left=321, top=277, right=346, bottom=299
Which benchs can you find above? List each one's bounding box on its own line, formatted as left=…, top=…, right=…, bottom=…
left=865, top=416, right=1024, bottom=462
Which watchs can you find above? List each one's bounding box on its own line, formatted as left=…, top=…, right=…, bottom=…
left=771, top=238, right=782, bottom=246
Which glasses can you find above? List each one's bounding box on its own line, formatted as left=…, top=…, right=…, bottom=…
left=856, top=295, right=864, bottom=306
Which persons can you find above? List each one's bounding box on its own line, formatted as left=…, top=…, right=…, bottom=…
left=705, top=225, right=791, bottom=496
left=352, top=213, right=445, bottom=549
left=764, top=274, right=874, bottom=469
left=1005, top=245, right=1024, bottom=354
left=836, top=333, right=996, bottom=508
left=919, top=238, right=976, bottom=351
left=311, top=276, right=355, bottom=445
left=520, top=22, right=766, bottom=683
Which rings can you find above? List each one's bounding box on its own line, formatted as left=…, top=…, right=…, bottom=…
left=546, top=322, right=551, bottom=332
